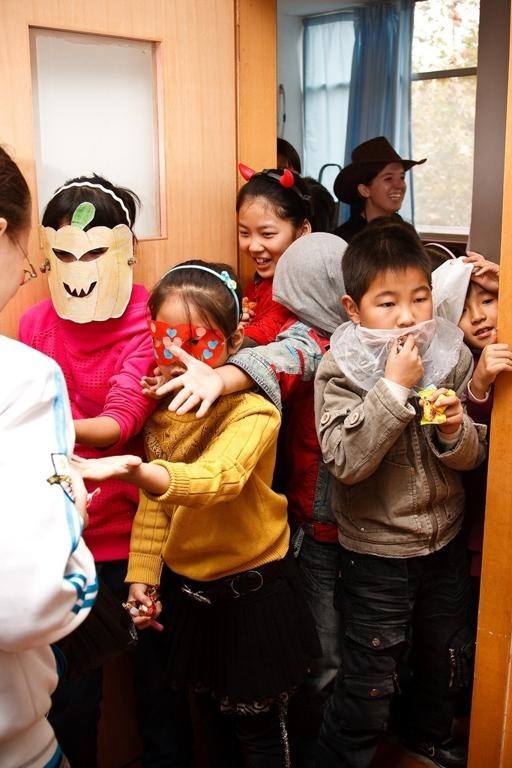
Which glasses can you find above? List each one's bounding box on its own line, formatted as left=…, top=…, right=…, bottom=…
left=11, top=233, right=37, bottom=286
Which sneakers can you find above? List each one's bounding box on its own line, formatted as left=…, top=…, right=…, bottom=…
left=406, top=739, right=466, bottom=768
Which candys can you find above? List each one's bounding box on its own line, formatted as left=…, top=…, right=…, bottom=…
left=419, top=388, right=455, bottom=426
left=123, top=583, right=164, bottom=632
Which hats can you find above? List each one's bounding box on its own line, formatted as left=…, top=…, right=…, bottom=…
left=333, top=136, right=427, bottom=204
